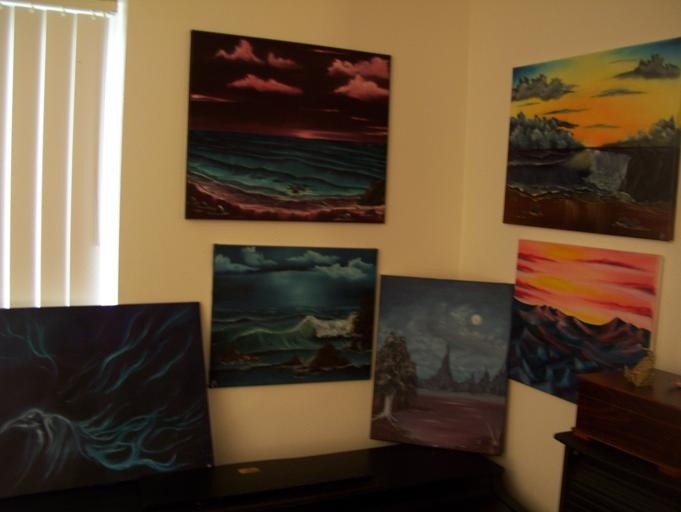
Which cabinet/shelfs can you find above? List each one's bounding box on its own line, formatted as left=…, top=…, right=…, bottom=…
left=553, top=431, right=681, bottom=512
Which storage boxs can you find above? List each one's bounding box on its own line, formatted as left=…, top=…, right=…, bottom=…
left=576, top=368, right=681, bottom=469
